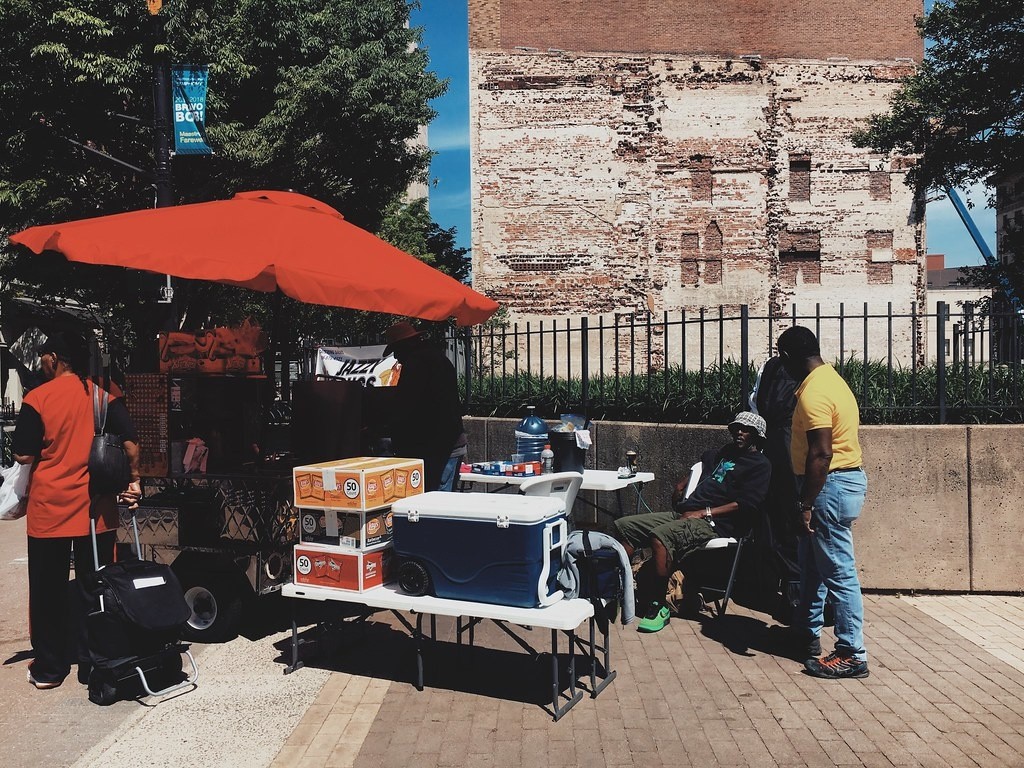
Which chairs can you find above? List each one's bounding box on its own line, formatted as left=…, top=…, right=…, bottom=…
left=673, top=491, right=801, bottom=622
left=117, top=475, right=293, bottom=551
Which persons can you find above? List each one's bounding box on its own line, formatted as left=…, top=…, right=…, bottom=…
left=11, top=333, right=141, bottom=689
left=613, top=412, right=772, bottom=633
left=750, top=354, right=805, bottom=465
left=778, top=326, right=869, bottom=678
left=360, top=321, right=468, bottom=492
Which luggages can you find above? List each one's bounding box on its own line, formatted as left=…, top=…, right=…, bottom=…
left=82, top=490, right=194, bottom=707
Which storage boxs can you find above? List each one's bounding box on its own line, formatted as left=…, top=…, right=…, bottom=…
left=392, top=492, right=568, bottom=606
left=294, top=543, right=399, bottom=594
left=294, top=456, right=424, bottom=513
left=298, top=506, right=394, bottom=551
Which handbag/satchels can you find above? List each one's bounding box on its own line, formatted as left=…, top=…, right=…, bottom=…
left=87, top=382, right=132, bottom=495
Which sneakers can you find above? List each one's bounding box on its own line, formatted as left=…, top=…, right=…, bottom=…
left=767, top=625, right=822, bottom=656
left=28, top=659, right=61, bottom=689
left=807, top=644, right=870, bottom=679
left=638, top=600, right=671, bottom=632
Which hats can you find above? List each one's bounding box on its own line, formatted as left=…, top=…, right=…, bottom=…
left=37, top=330, right=92, bottom=362
left=728, top=411, right=768, bottom=440
left=382, top=321, right=423, bottom=356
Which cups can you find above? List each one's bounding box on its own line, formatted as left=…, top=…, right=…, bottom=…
left=511, top=454, right=525, bottom=462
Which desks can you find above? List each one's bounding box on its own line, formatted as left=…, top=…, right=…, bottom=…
left=280, top=573, right=617, bottom=722
left=452, top=465, right=655, bottom=520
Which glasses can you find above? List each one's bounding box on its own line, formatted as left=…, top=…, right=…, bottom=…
left=39, top=351, right=51, bottom=357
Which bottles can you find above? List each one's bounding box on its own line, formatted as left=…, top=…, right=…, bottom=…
left=515, top=405, right=549, bottom=461
left=541, top=444, right=554, bottom=475
left=626, top=451, right=637, bottom=475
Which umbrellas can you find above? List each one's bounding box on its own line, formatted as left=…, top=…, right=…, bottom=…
left=8, top=190, right=500, bottom=394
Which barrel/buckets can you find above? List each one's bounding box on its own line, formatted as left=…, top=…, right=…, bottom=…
left=547, top=430, right=587, bottom=475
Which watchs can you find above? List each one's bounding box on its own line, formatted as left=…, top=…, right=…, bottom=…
left=705, top=507, right=712, bottom=522
left=794, top=501, right=815, bottom=512
